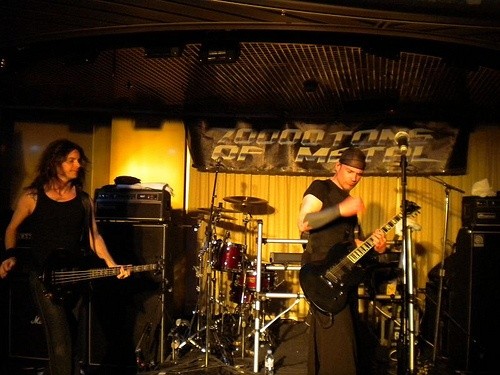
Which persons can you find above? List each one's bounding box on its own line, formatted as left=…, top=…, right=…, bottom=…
left=299, top=148, right=387, bottom=375
left=0, top=141, right=131, bottom=375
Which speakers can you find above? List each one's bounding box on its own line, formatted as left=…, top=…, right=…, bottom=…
left=85, top=221, right=185, bottom=368
left=0, top=238, right=50, bottom=363
left=450, top=227, right=500, bottom=375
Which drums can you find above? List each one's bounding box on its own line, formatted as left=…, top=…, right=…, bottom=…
left=210, top=240, right=246, bottom=274
left=228, top=267, right=278, bottom=303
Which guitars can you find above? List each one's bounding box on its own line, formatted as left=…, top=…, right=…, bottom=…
left=20, top=253, right=165, bottom=318
left=298, top=198, right=420, bottom=315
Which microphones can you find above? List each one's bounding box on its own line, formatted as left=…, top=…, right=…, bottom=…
left=216, top=157, right=223, bottom=167
left=394, top=131, right=409, bottom=154
left=421, top=171, right=446, bottom=186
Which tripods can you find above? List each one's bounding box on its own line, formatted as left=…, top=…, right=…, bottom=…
left=172, top=164, right=255, bottom=367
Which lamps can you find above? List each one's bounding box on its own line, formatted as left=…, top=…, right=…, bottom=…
left=197, top=43, right=242, bottom=65
left=143, top=44, right=183, bottom=58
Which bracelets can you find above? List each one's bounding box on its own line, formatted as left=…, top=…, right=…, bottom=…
left=4, top=248, right=16, bottom=260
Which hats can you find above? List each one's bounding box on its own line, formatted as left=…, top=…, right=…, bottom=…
left=339, top=147, right=366, bottom=170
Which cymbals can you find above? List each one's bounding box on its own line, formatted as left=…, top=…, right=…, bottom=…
left=197, top=205, right=240, bottom=220
left=221, top=195, right=268, bottom=206
